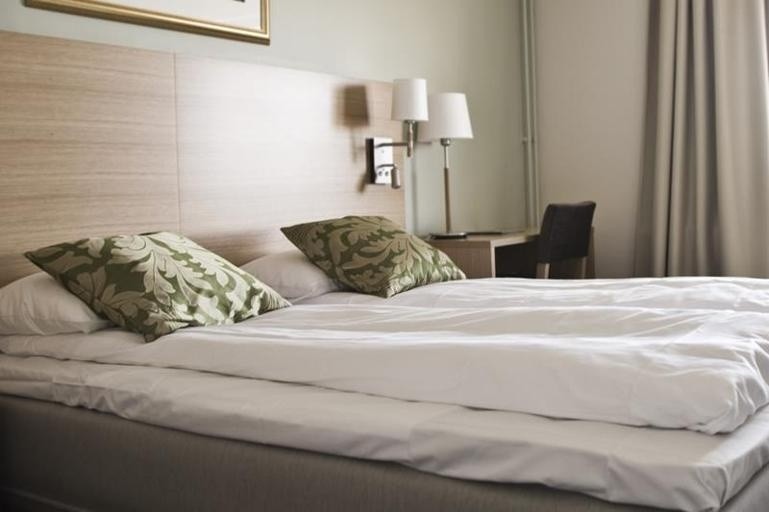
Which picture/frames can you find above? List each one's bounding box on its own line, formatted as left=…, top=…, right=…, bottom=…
left=23, top=0, right=272, bottom=46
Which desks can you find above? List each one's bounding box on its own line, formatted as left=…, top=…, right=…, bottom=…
left=426, top=226, right=598, bottom=278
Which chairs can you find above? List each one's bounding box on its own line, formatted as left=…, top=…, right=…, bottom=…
left=535, top=201, right=597, bottom=279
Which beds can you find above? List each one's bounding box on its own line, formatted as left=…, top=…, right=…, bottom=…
left=2, top=29, right=769, bottom=509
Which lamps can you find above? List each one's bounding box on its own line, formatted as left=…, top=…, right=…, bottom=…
left=364, top=78, right=430, bottom=189
left=416, top=92, right=474, bottom=240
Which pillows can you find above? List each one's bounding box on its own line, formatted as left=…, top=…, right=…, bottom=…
left=0, top=270, right=119, bottom=335
left=279, top=215, right=468, bottom=299
left=20, top=229, right=294, bottom=344
left=240, top=247, right=341, bottom=304
